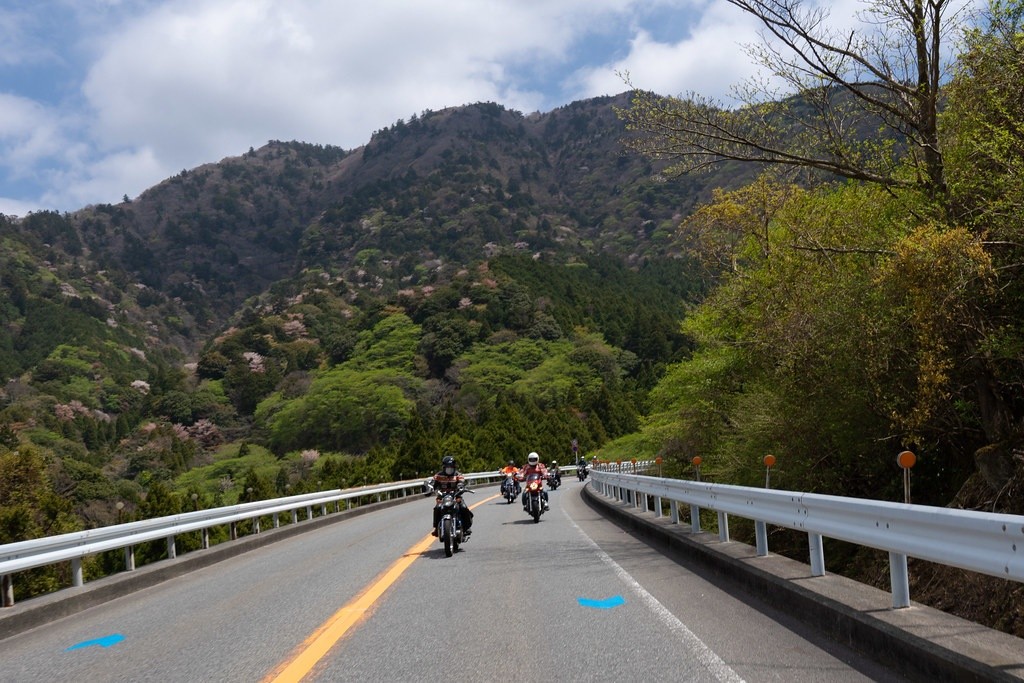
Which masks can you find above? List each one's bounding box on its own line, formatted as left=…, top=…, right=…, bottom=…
left=446, top=467, right=454, bottom=474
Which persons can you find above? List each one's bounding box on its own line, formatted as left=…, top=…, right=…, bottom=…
left=497, top=460, right=523, bottom=499
left=425, top=455, right=474, bottom=537
left=576, top=455, right=589, bottom=478
left=544, top=460, right=562, bottom=486
left=591, top=455, right=600, bottom=467
left=514, top=452, right=555, bottom=512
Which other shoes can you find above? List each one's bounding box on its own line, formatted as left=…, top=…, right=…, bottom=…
left=524, top=506, right=528, bottom=510
left=463, top=530, right=471, bottom=535
left=431, top=528, right=436, bottom=535
left=543, top=502, right=549, bottom=510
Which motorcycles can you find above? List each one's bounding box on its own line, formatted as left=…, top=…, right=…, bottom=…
left=516, top=472, right=552, bottom=523
left=500, top=470, right=521, bottom=503
left=576, top=463, right=587, bottom=482
left=546, top=469, right=558, bottom=490
left=422, top=480, right=476, bottom=558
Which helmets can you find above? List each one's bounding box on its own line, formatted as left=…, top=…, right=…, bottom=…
left=442, top=456, right=455, bottom=466
left=527, top=451, right=539, bottom=465
left=551, top=460, right=556, bottom=467
left=506, top=459, right=514, bottom=466
